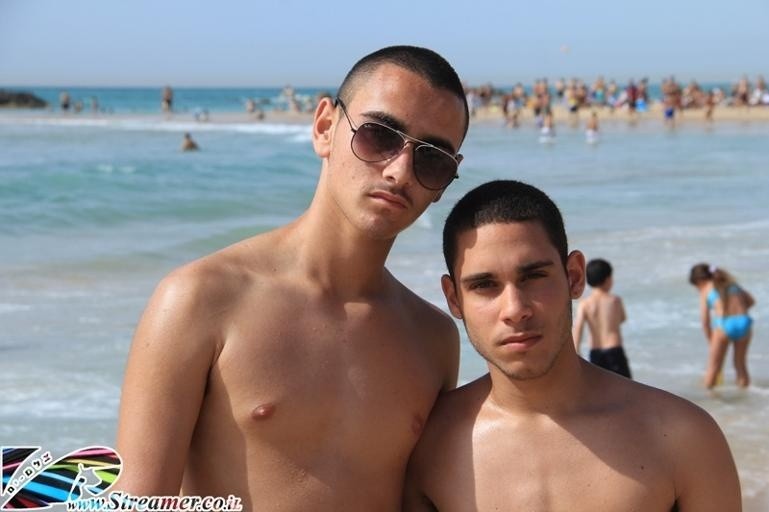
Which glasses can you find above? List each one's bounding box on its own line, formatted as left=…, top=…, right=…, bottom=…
left=336, top=95, right=459, bottom=191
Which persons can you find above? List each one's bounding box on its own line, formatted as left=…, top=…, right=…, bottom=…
left=182, top=132, right=200, bottom=153
left=687, top=259, right=756, bottom=393
left=60, top=89, right=71, bottom=113
left=398, top=179, right=745, bottom=512
left=159, top=82, right=174, bottom=122
left=571, top=257, right=633, bottom=379
left=98, top=44, right=474, bottom=511
left=236, top=68, right=769, bottom=145
